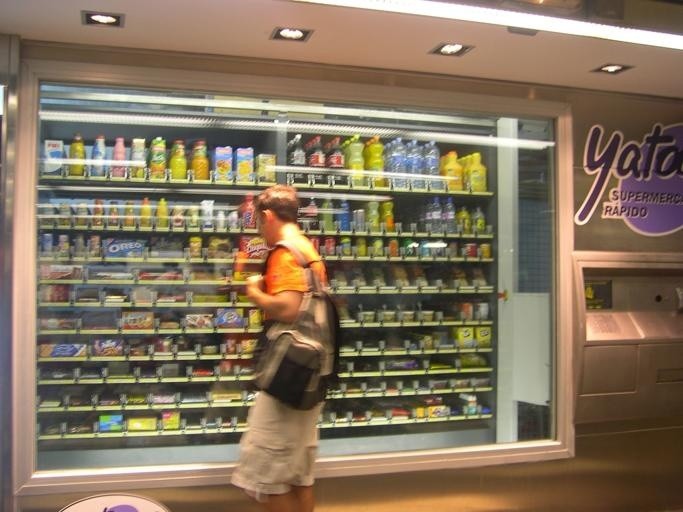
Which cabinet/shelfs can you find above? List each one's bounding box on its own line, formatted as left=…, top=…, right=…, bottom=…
left=281, top=119, right=493, bottom=432
left=31, top=112, right=281, bottom=447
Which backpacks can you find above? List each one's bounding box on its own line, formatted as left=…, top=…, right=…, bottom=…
left=248, top=240, right=341, bottom=412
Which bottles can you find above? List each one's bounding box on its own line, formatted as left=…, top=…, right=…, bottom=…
left=288, top=134, right=487, bottom=190
left=70, top=132, right=209, bottom=182
left=90, top=197, right=169, bottom=230
left=418, top=195, right=485, bottom=234
left=306, top=197, right=395, bottom=232
left=242, top=193, right=256, bottom=227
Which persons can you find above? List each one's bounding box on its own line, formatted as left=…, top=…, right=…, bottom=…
left=228, top=185, right=329, bottom=512
